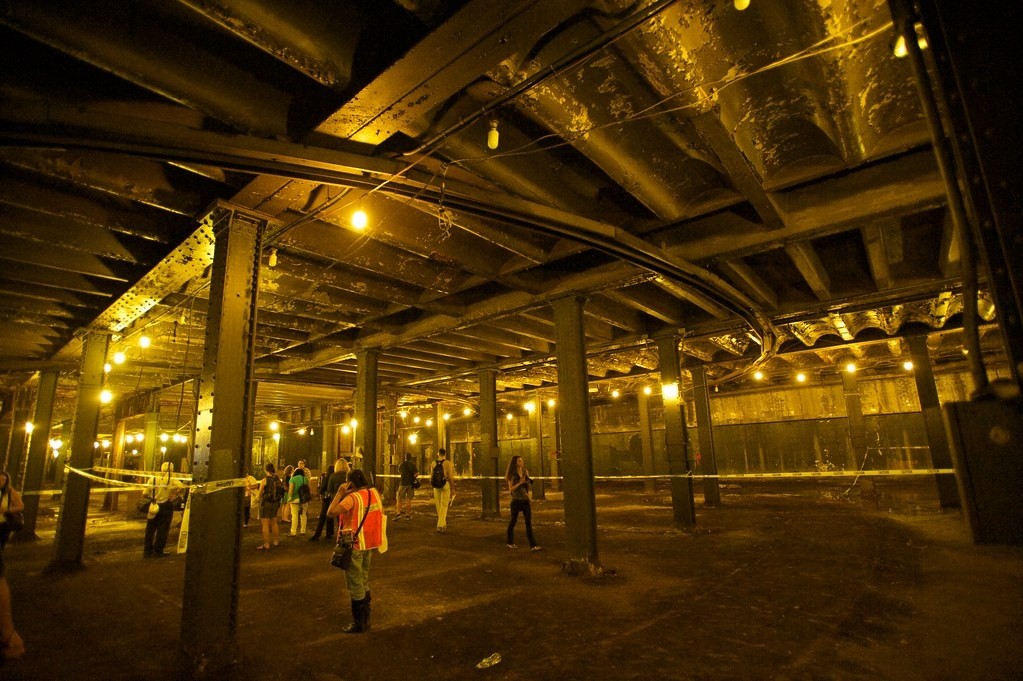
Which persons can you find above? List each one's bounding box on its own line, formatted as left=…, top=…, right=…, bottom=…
left=392, top=453, right=419, bottom=520
left=245, top=459, right=353, bottom=547
left=141, top=462, right=190, bottom=558
left=507, top=456, right=542, bottom=552
left=0, top=471, right=25, bottom=660
left=431, top=449, right=454, bottom=532
left=328, top=469, right=383, bottom=632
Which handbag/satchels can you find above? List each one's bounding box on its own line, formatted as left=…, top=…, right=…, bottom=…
left=298, top=485, right=311, bottom=503
left=331, top=542, right=353, bottom=570
left=7, top=512, right=25, bottom=531
left=137, top=498, right=154, bottom=513
left=413, top=481, right=421, bottom=488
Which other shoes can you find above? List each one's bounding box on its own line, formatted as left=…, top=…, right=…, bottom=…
left=404, top=515, right=412, bottom=520
left=436, top=526, right=446, bottom=532
left=257, top=545, right=271, bottom=550
left=529, top=546, right=543, bottom=551
left=153, top=552, right=171, bottom=556
left=300, top=533, right=305, bottom=535
left=393, top=513, right=402, bottom=520
left=282, top=519, right=293, bottom=523
left=287, top=534, right=295, bottom=537
left=324, top=535, right=332, bottom=538
left=273, top=542, right=280, bottom=547
left=507, top=544, right=518, bottom=548
left=243, top=525, right=248, bottom=528
left=308, top=537, right=319, bottom=541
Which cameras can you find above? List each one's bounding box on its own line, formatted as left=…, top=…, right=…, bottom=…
left=524, top=475, right=533, bottom=484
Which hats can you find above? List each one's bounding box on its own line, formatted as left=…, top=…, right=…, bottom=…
left=147, top=502, right=160, bottom=519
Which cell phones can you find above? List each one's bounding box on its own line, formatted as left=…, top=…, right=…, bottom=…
left=348, top=485, right=353, bottom=490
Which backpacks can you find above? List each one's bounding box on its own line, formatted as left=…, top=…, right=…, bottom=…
left=431, top=460, right=446, bottom=488
left=266, top=477, right=281, bottom=503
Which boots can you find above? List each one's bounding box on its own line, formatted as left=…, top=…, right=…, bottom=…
left=342, top=591, right=371, bottom=632
